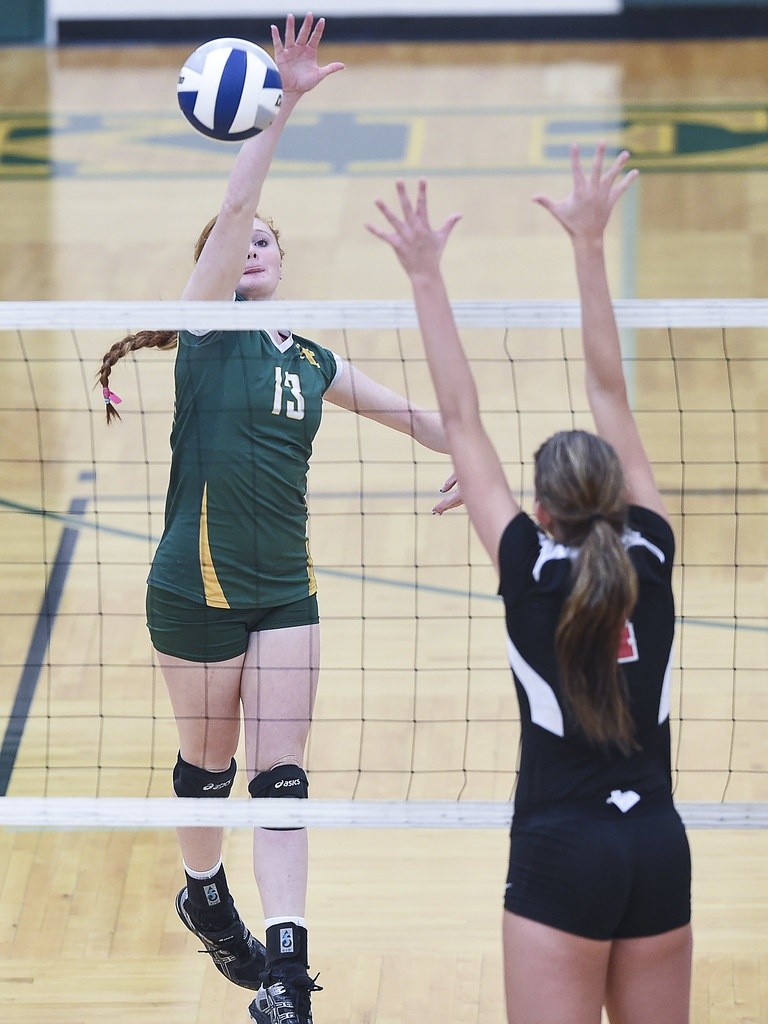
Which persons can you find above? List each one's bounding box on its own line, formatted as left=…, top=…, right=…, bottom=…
left=361, top=139, right=698, bottom=1024
left=93, top=11, right=465, bottom=1024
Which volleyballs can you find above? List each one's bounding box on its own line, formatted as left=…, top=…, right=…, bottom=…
left=176, top=38, right=283, bottom=140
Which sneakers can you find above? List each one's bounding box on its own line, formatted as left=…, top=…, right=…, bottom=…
left=248, top=970, right=323, bottom=1024
left=174, top=886, right=266, bottom=992
